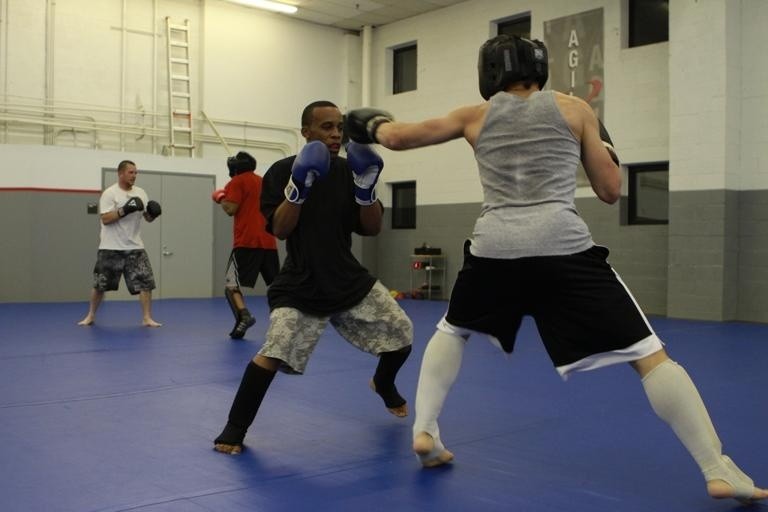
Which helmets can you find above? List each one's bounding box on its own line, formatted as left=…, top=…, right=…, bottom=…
left=477, top=33, right=550, bottom=101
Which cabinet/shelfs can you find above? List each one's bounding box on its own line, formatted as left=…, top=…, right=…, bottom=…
left=409, top=254, right=447, bottom=302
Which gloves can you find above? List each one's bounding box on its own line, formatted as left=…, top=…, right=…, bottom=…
left=344, top=140, right=385, bottom=206
left=118, top=196, right=144, bottom=217
left=347, top=107, right=394, bottom=145
left=147, top=200, right=161, bottom=219
left=211, top=188, right=226, bottom=204
left=283, top=141, right=331, bottom=205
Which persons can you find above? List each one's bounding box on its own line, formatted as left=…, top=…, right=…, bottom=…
left=212, top=151, right=280, bottom=340
left=214, top=100, right=415, bottom=456
left=77, top=160, right=162, bottom=328
left=341, top=34, right=768, bottom=502
